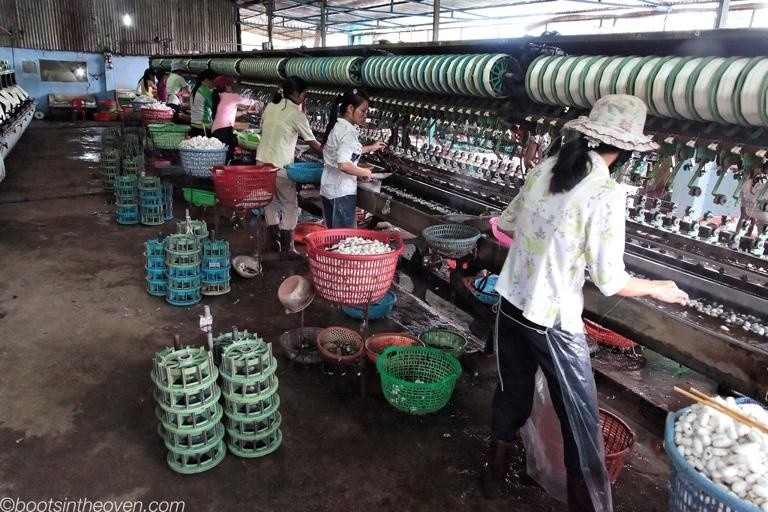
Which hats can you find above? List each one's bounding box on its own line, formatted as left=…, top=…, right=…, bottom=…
left=214, top=76, right=232, bottom=89
left=561, top=94, right=660, bottom=153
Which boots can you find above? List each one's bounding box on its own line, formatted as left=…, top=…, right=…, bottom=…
left=281, top=230, right=306, bottom=259
left=269, top=224, right=282, bottom=253
left=479, top=439, right=504, bottom=500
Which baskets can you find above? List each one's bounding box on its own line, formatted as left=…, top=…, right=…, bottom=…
left=178, top=131, right=324, bottom=209
left=664, top=397, right=768, bottom=512
left=93, top=98, right=191, bottom=150
left=279, top=223, right=481, bottom=415
left=541, top=407, right=636, bottom=484
left=584, top=317, right=638, bottom=347
left=474, top=276, right=504, bottom=304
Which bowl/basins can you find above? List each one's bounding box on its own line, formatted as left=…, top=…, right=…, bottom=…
left=489, top=217, right=512, bottom=244
left=94, top=112, right=111, bottom=122
left=237, top=136, right=258, bottom=150
left=294, top=221, right=328, bottom=243
left=278, top=275, right=316, bottom=313
left=471, top=276, right=500, bottom=305
left=182, top=187, right=219, bottom=208
left=283, top=162, right=324, bottom=184
left=365, top=333, right=425, bottom=363
left=418, top=329, right=469, bottom=358
left=233, top=255, right=262, bottom=277
left=343, top=290, right=398, bottom=320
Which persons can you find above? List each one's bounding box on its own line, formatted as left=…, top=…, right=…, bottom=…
left=155, top=68, right=168, bottom=102
left=210, top=74, right=257, bottom=165
left=487, top=95, right=694, bottom=510
left=165, top=60, right=192, bottom=118
left=319, top=87, right=388, bottom=230
left=138, top=70, right=157, bottom=100
left=256, top=75, right=324, bottom=260
left=188, top=68, right=216, bottom=138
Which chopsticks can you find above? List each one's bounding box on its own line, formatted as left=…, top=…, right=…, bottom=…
left=672, top=385, right=768, bottom=434
